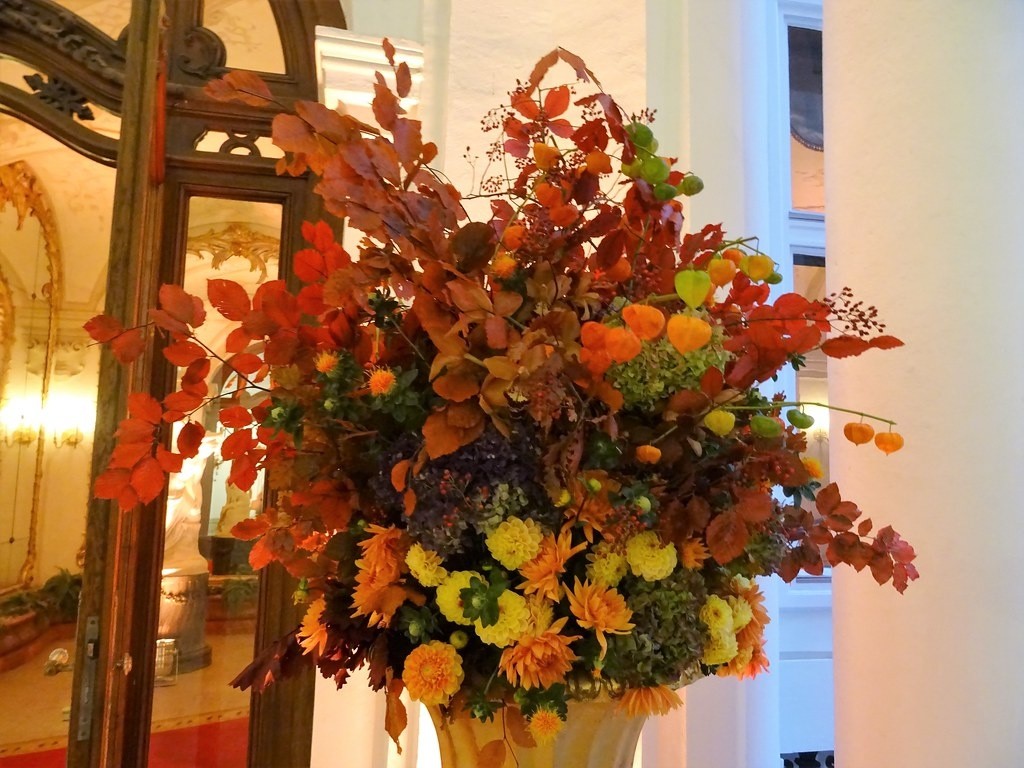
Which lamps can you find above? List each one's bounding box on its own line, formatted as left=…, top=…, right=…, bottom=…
left=40, top=647, right=74, bottom=676
left=155, top=638, right=180, bottom=689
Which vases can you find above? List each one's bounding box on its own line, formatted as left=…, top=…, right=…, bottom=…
left=423, top=684, right=652, bottom=767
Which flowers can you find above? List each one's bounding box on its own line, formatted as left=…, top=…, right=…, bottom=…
left=84, top=32, right=918, bottom=767
